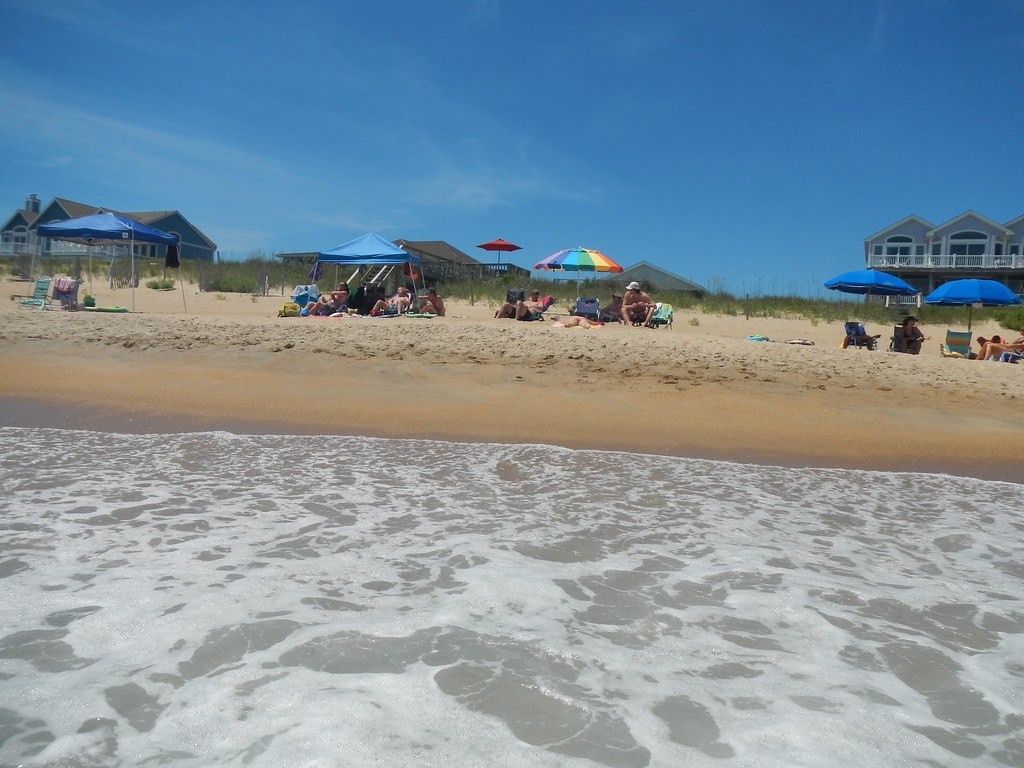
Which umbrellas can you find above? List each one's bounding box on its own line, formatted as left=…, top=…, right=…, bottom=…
left=925, top=279, right=1020, bottom=331
left=534, top=246, right=625, bottom=297
left=824, top=269, right=917, bottom=324
left=476, top=239, right=523, bottom=263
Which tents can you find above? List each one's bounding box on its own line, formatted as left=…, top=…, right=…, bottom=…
left=27, top=211, right=187, bottom=313
left=307, top=231, right=427, bottom=313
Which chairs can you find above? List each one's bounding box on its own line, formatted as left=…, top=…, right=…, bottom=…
left=380, top=293, right=411, bottom=316
left=649, top=303, right=673, bottom=329
left=536, top=297, right=553, bottom=320
left=570, top=297, right=601, bottom=322
left=318, top=291, right=351, bottom=315
left=939, top=329, right=973, bottom=359
left=990, top=348, right=1024, bottom=363
left=844, top=320, right=878, bottom=352
left=888, top=324, right=918, bottom=353
left=17, top=278, right=84, bottom=311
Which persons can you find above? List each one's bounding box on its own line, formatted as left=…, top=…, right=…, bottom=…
left=495, top=282, right=656, bottom=330
left=843, top=317, right=1024, bottom=360
left=302, top=281, right=446, bottom=317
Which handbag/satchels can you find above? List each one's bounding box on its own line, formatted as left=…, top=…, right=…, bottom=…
left=278, top=302, right=301, bottom=317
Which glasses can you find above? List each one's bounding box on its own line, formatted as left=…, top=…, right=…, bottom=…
left=339, top=284, right=343, bottom=287
left=1019, top=329, right=1024, bottom=331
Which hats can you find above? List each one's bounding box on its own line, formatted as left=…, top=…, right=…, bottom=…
left=531, top=289, right=540, bottom=295
left=427, top=287, right=436, bottom=292
left=907, top=316, right=918, bottom=321
left=611, top=291, right=623, bottom=297
left=626, top=282, right=640, bottom=290
